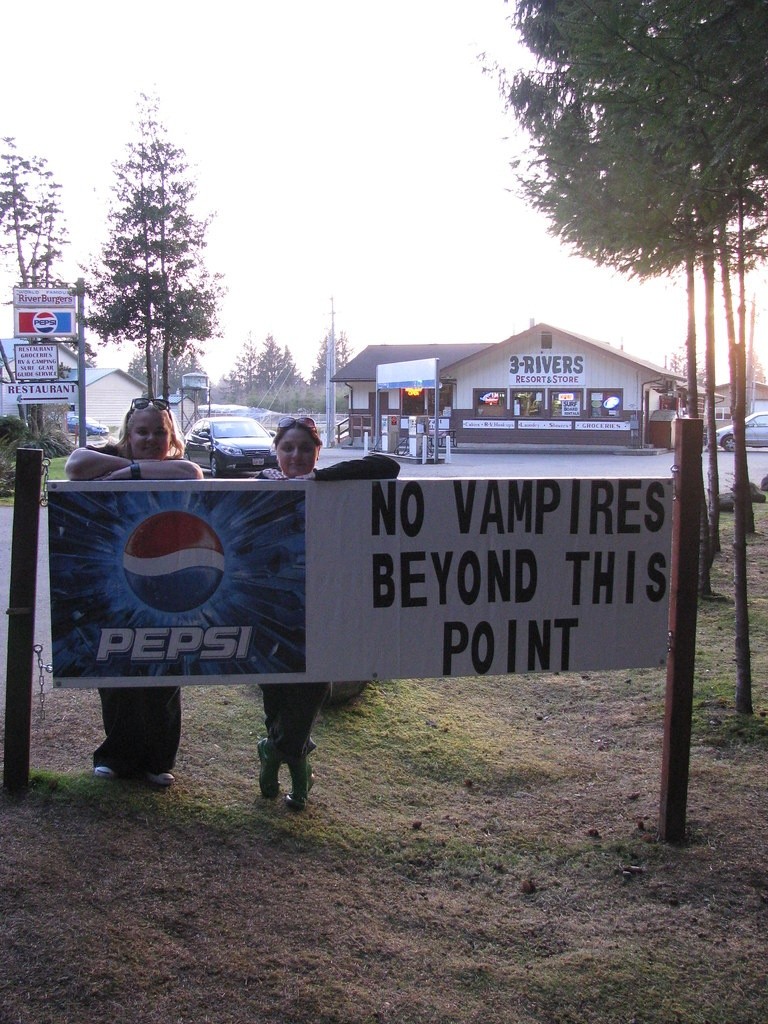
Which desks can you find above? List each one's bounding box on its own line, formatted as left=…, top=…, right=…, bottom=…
left=429, top=429, right=457, bottom=446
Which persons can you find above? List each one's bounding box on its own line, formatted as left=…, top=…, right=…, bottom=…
left=250, top=417, right=402, bottom=805
left=63, top=396, right=204, bottom=784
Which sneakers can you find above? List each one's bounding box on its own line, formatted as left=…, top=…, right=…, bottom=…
left=257, top=738, right=280, bottom=798
left=285, top=773, right=315, bottom=809
left=95, top=760, right=119, bottom=779
left=143, top=766, right=175, bottom=785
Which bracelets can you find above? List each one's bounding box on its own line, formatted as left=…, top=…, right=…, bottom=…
left=130, top=462, right=141, bottom=480
left=131, top=458, right=135, bottom=464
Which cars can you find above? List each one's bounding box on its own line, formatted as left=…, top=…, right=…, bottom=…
left=183, top=417, right=283, bottom=478
left=68, top=416, right=109, bottom=436
left=716, top=412, right=768, bottom=451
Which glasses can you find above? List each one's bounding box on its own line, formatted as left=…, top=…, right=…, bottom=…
left=277, top=417, right=317, bottom=433
left=130, top=398, right=169, bottom=416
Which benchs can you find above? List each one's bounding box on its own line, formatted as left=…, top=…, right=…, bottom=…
left=428, top=435, right=458, bottom=447
